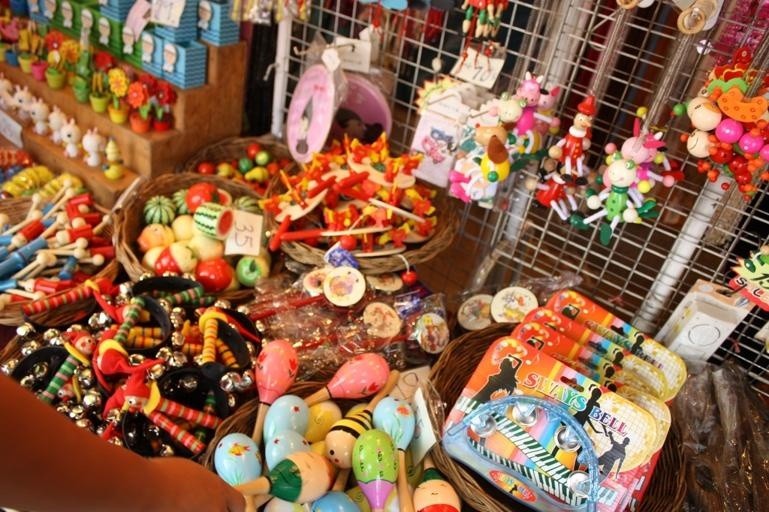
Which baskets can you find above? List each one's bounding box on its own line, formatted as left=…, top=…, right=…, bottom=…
left=120, top=137, right=462, bottom=299
left=199, top=381, right=457, bottom=512
left=424, top=320, right=688, bottom=512
left=4, top=296, right=258, bottom=472
left=1, top=190, right=118, bottom=330
